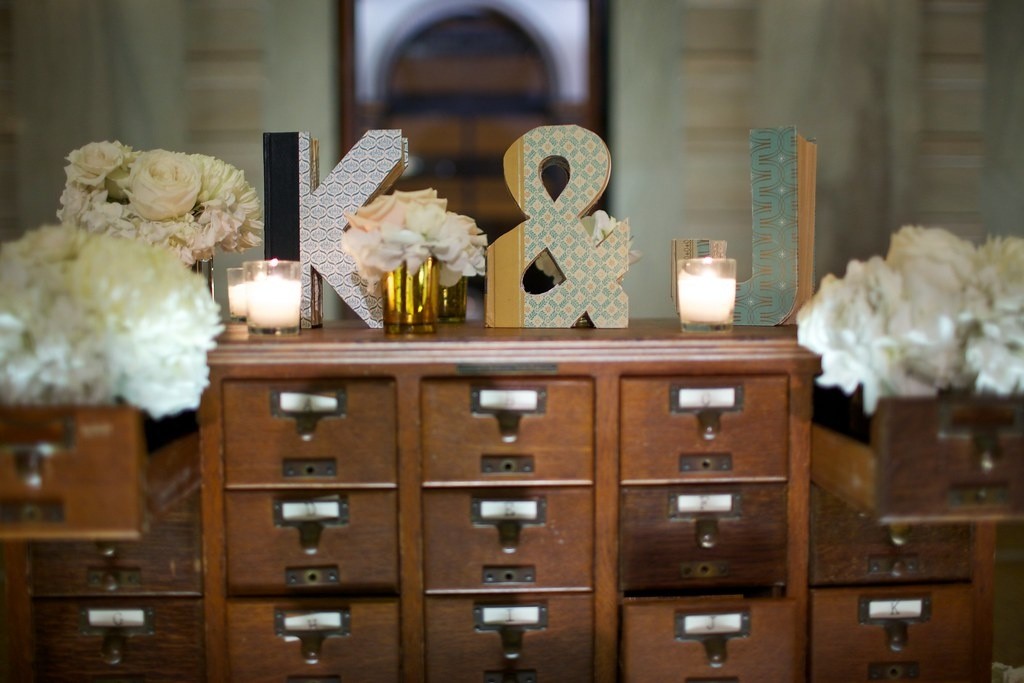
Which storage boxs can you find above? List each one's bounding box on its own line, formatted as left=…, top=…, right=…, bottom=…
left=0, top=311, right=1023, bottom=683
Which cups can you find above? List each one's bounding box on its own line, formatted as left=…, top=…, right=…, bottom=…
left=243, top=260, right=303, bottom=337
left=226, top=268, right=250, bottom=323
left=676, top=259, right=736, bottom=335
